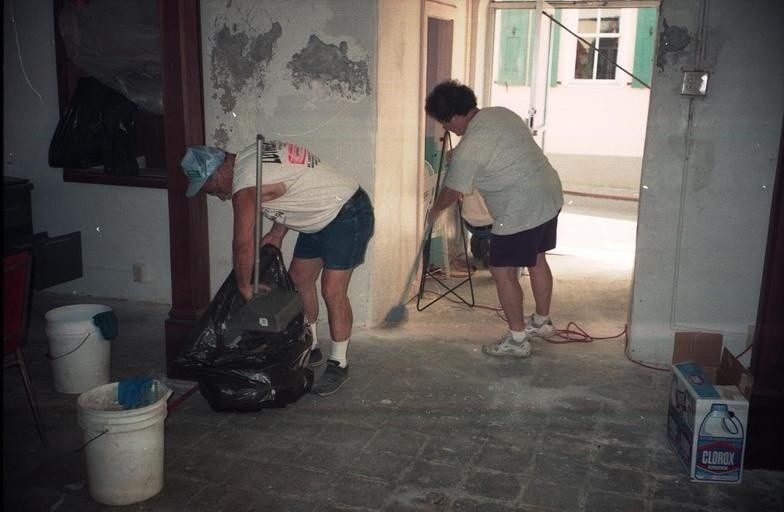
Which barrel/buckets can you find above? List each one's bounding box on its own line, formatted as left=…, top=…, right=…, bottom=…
left=44, top=304, right=113, bottom=394
left=76, top=381, right=168, bottom=505
left=695, top=404, right=745, bottom=482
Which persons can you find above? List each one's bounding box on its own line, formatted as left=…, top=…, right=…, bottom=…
left=426, top=81, right=564, bottom=359
left=181, top=141, right=374, bottom=396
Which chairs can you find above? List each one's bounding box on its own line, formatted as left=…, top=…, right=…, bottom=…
left=423, top=160, right=451, bottom=279
left=1, top=253, right=48, bottom=447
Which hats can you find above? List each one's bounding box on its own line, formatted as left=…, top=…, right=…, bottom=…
left=180, top=145, right=226, bottom=199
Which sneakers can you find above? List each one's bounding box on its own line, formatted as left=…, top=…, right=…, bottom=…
left=482, top=331, right=532, bottom=357
left=524, top=313, right=554, bottom=336
left=309, top=347, right=321, bottom=365
left=315, top=359, right=351, bottom=396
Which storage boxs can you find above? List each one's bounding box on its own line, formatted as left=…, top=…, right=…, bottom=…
left=667, top=328, right=753, bottom=486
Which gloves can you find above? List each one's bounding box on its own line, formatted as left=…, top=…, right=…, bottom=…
left=118, top=377, right=153, bottom=409
